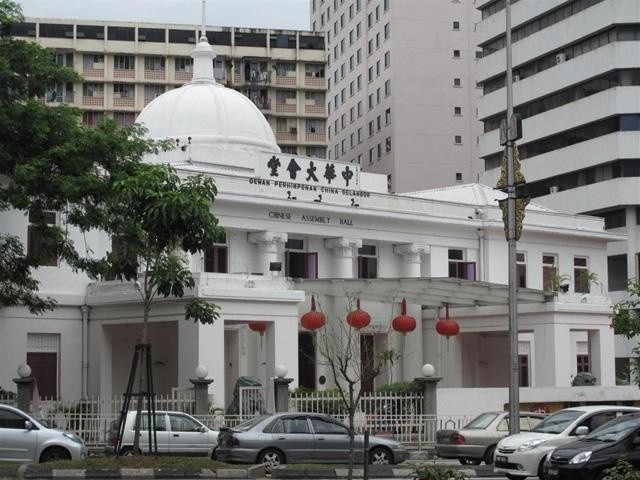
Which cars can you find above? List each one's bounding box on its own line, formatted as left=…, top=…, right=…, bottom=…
left=435, top=405, right=639, bottom=480
left=215, top=412, right=405, bottom=474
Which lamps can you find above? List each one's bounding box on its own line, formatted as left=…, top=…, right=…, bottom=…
left=17, top=363, right=32, bottom=378
left=422, top=364, right=435, bottom=377
left=274, top=364, right=289, bottom=378
left=194, top=366, right=209, bottom=379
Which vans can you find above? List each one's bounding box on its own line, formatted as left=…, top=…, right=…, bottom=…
left=107, top=410, right=218, bottom=458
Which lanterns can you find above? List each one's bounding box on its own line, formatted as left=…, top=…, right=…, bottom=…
left=436, top=304, right=460, bottom=352
left=347, top=298, right=371, bottom=343
left=247, top=323, right=268, bottom=352
left=301, top=294, right=326, bottom=344
left=392, top=298, right=416, bottom=351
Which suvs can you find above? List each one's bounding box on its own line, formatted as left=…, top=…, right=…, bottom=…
left=0, top=403, right=88, bottom=465
